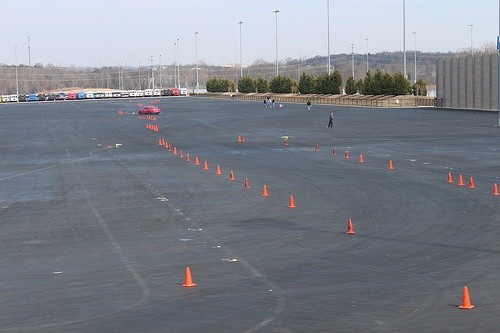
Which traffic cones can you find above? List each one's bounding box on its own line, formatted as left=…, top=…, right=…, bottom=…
left=345, top=218, right=355, bottom=234
left=288, top=195, right=296, bottom=208
left=186, top=154, right=190, bottom=161
left=215, top=165, right=222, bottom=175
left=236, top=134, right=363, bottom=163
left=145, top=123, right=159, bottom=132
left=158, top=139, right=183, bottom=158
left=182, top=266, right=196, bottom=287
left=446, top=172, right=475, bottom=188
left=203, top=161, right=209, bottom=170
left=492, top=183, right=499, bottom=196
left=229, top=170, right=235, bottom=180
left=194, top=156, right=200, bottom=165
left=261, top=185, right=269, bottom=197
left=458, top=286, right=476, bottom=309
left=388, top=159, right=394, bottom=170
left=244, top=178, right=250, bottom=189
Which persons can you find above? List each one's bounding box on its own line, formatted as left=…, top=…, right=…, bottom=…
left=328, top=110, right=334, bottom=128
left=264, top=94, right=275, bottom=109
left=306, top=101, right=312, bottom=111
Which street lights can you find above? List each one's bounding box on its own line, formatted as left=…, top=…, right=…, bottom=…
left=469, top=24, right=473, bottom=50
left=239, top=20, right=244, bottom=77
left=412, top=31, right=417, bottom=83
left=195, top=31, right=199, bottom=96
left=275, top=9, right=279, bottom=77
left=365, top=38, right=369, bottom=72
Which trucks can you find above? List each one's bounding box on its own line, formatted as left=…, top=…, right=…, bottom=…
left=0, top=87, right=188, bottom=103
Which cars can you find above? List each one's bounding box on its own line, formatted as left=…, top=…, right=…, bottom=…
left=138, top=106, right=160, bottom=115
